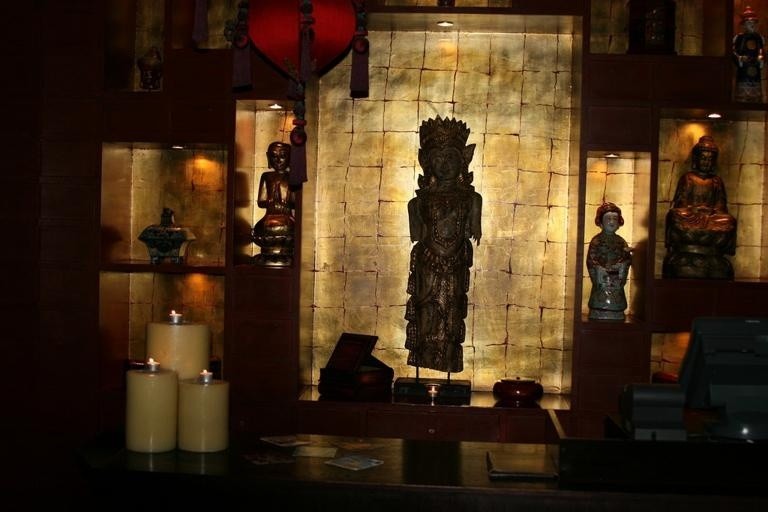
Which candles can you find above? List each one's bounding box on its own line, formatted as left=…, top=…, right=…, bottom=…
left=178, top=368, right=231, bottom=455
left=145, top=308, right=212, bottom=382
left=126, top=355, right=179, bottom=454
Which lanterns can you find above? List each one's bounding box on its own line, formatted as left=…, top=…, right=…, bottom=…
left=231, top=0, right=369, bottom=184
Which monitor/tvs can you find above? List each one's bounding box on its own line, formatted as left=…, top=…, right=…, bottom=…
left=678, top=316, right=768, bottom=439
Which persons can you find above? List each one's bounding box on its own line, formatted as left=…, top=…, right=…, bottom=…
left=586, top=202, right=633, bottom=319
left=732, top=5, right=765, bottom=103
left=404, top=114, right=482, bottom=373
left=666, top=136, right=737, bottom=255
left=254, top=142, right=297, bottom=236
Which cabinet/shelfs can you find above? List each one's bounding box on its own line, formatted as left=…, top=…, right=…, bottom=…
left=38, top=0, right=766, bottom=448
